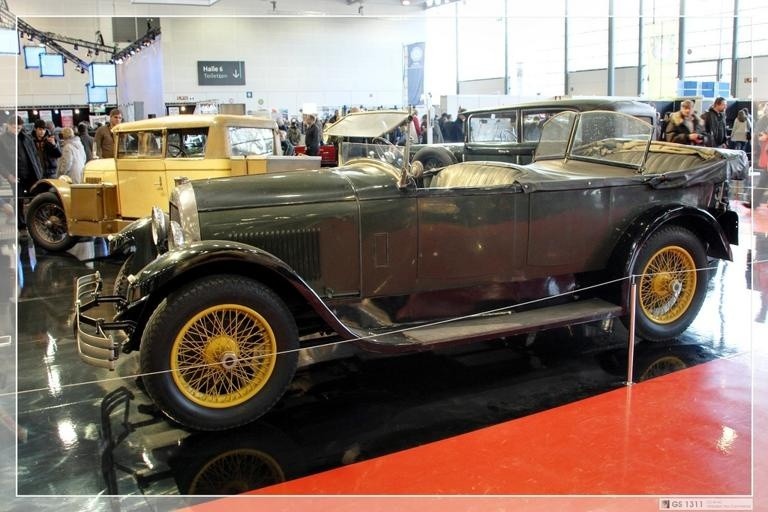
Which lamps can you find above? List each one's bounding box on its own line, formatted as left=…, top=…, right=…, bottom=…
left=0, top=0, right=166, bottom=107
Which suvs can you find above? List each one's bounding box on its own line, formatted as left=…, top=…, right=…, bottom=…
left=93, top=342, right=705, bottom=500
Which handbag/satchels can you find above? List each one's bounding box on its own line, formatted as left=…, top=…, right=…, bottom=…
left=45, top=156, right=58, bottom=175
left=747, top=130, right=751, bottom=141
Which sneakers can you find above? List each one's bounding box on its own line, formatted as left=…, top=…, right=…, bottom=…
left=19, top=228, right=28, bottom=237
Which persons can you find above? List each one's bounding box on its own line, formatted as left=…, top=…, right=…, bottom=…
left=751, top=232, right=768, bottom=324
left=8, top=237, right=60, bottom=350
left=663, top=96, right=767, bottom=210
left=271, top=104, right=465, bottom=157
left=0, top=109, right=124, bottom=240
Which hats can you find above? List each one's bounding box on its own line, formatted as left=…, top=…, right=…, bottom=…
left=34, top=119, right=50, bottom=130
